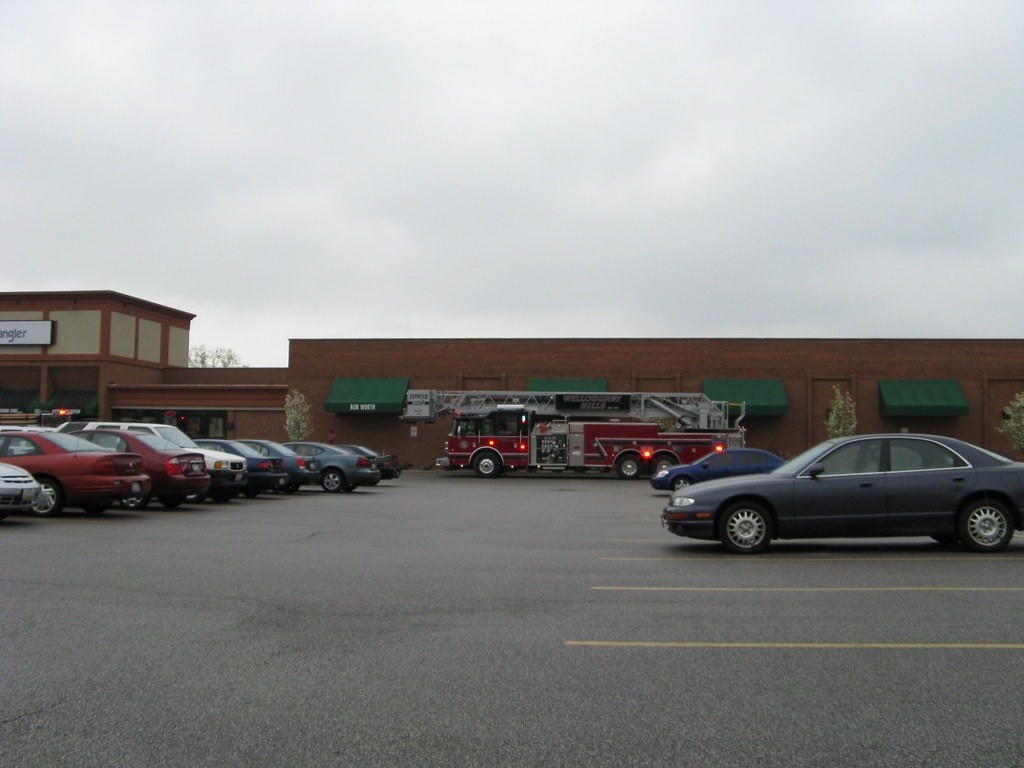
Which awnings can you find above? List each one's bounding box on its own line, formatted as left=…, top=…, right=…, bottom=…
left=527, top=377, right=608, bottom=391
left=324, top=377, right=409, bottom=413
left=879, top=379, right=970, bottom=417
left=704, top=379, right=788, bottom=416
left=27, top=391, right=99, bottom=416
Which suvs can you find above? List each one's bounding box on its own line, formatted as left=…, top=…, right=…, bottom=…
left=55, top=421, right=249, bottom=503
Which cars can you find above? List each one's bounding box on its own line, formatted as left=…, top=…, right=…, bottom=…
left=1, top=431, right=152, bottom=517
left=662, top=432, right=1024, bottom=556
left=193, top=439, right=291, bottom=498
left=260, top=442, right=382, bottom=493
left=64, top=429, right=212, bottom=511
left=650, top=448, right=792, bottom=494
left=329, top=444, right=399, bottom=486
left=0, top=462, right=42, bottom=521
left=230, top=438, right=322, bottom=495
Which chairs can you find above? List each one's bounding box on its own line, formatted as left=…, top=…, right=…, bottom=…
left=859, top=447, right=880, bottom=472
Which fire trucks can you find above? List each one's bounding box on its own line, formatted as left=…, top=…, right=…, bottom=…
left=398, top=389, right=748, bottom=480
